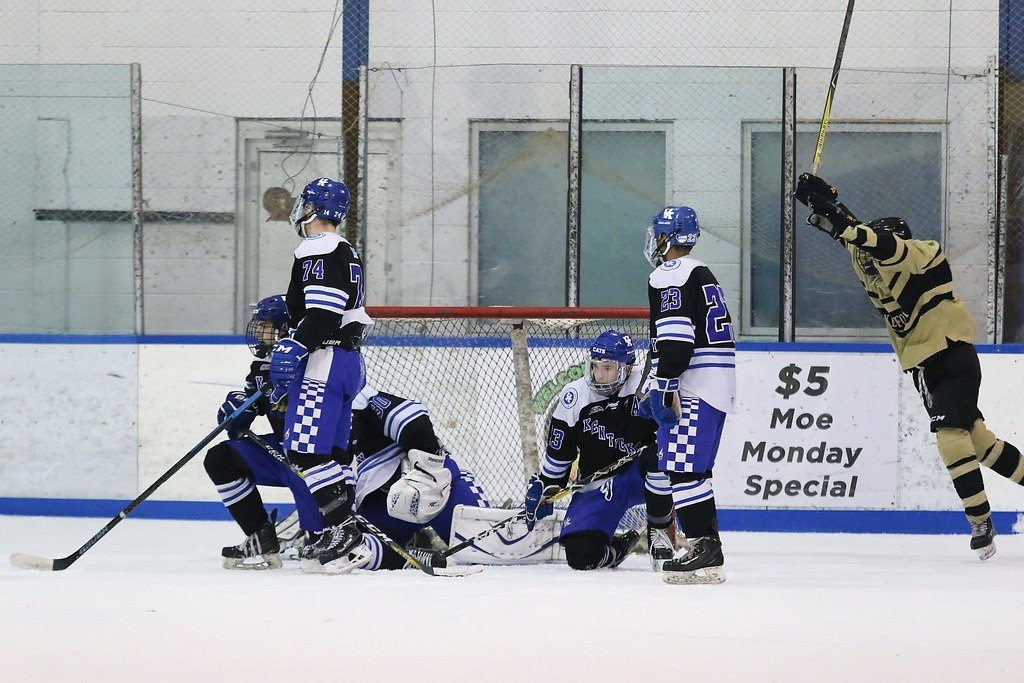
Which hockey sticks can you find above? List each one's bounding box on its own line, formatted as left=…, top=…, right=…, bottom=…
left=239, top=426, right=486, bottom=577
left=423, top=443, right=652, bottom=559
left=810, top=0, right=856, bottom=178
left=633, top=350, right=652, bottom=400
left=8, top=380, right=274, bottom=573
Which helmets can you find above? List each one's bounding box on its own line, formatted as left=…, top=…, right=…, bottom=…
left=865, top=217, right=912, bottom=242
left=244, top=294, right=291, bottom=358
left=586, top=329, right=636, bottom=395
left=643, top=206, right=700, bottom=268
left=288, top=177, right=351, bottom=238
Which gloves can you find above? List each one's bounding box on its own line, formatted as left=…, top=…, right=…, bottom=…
left=270, top=338, right=308, bottom=389
left=217, top=391, right=259, bottom=439
left=793, top=173, right=838, bottom=211
left=526, top=477, right=560, bottom=532
left=639, top=388, right=655, bottom=421
left=648, top=373, right=683, bottom=426
left=806, top=192, right=850, bottom=239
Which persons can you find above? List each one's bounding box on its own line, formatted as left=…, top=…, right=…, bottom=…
left=203, top=295, right=448, bottom=572
left=795, top=172, right=1024, bottom=561
left=636, top=205, right=737, bottom=586
left=525, top=329, right=675, bottom=572
left=267, top=177, right=373, bottom=577
left=356, top=384, right=489, bottom=549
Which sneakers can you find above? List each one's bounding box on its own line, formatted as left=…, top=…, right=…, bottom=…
left=608, top=528, right=645, bottom=567
left=320, top=513, right=373, bottom=576
left=662, top=536, right=726, bottom=584
left=221, top=507, right=283, bottom=571
left=645, top=520, right=673, bottom=571
left=970, top=518, right=997, bottom=561
left=299, top=525, right=337, bottom=573
